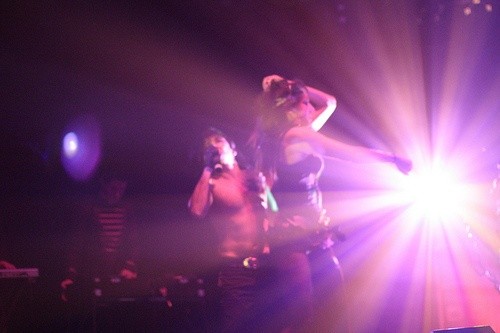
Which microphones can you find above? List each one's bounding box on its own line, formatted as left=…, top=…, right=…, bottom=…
left=213, top=153, right=222, bottom=172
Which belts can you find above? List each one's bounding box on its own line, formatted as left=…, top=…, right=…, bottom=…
left=219, top=255, right=270, bottom=269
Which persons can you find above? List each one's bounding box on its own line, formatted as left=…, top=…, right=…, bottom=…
left=61, top=161, right=138, bottom=277
left=189, top=126, right=280, bottom=264
left=246, top=75, right=418, bottom=333
